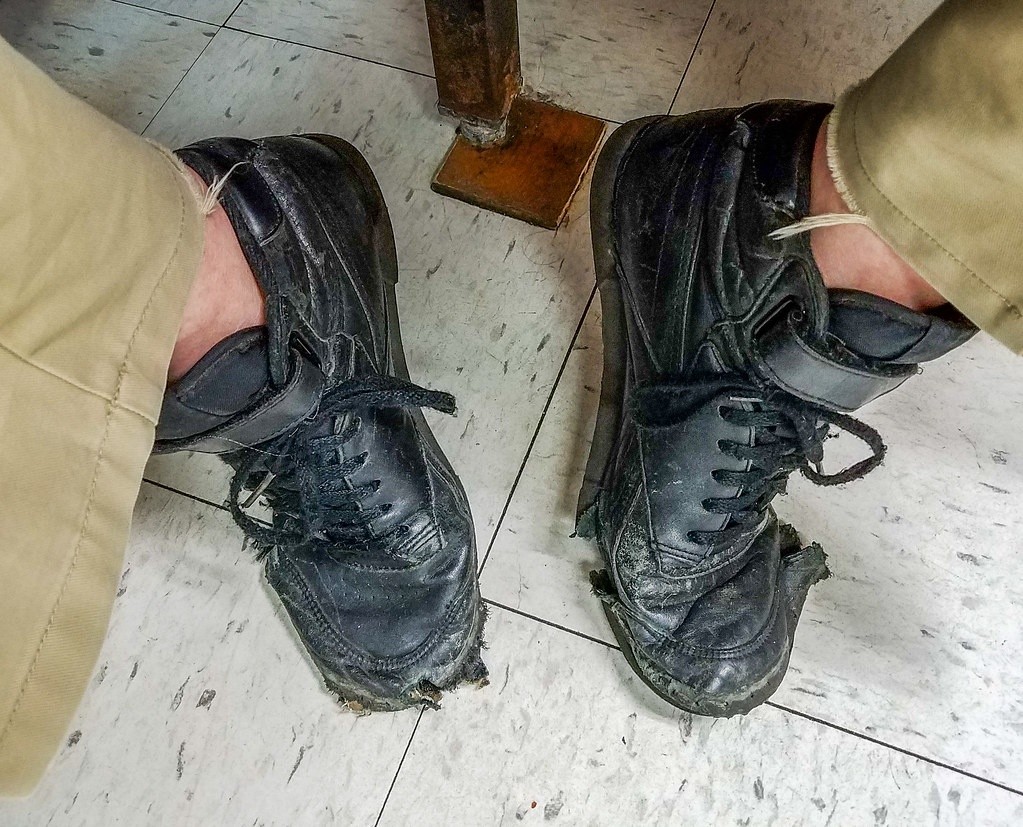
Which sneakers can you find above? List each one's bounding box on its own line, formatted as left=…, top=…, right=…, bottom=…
left=154, top=133, right=489, bottom=713
left=592, top=97, right=977, bottom=717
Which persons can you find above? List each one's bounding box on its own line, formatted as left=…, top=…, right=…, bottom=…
left=0, top=0, right=1023, bottom=790
